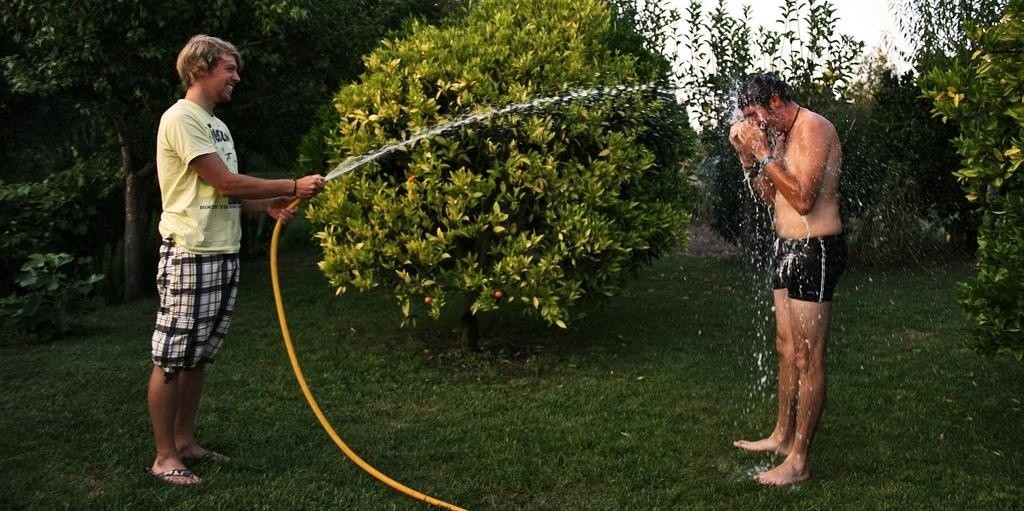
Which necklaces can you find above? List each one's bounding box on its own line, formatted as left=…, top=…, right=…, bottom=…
left=196, top=102, right=215, bottom=117
left=784, top=105, right=801, bottom=148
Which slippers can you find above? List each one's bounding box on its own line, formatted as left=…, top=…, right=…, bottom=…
left=152, top=448, right=231, bottom=486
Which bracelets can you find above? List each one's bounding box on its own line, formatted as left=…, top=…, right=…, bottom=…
left=292, top=179, right=298, bottom=196
left=741, top=164, right=759, bottom=179
left=760, top=155, right=775, bottom=169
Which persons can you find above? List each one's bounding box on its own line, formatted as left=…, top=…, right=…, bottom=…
left=727, top=74, right=849, bottom=487
left=146, top=34, right=325, bottom=488
